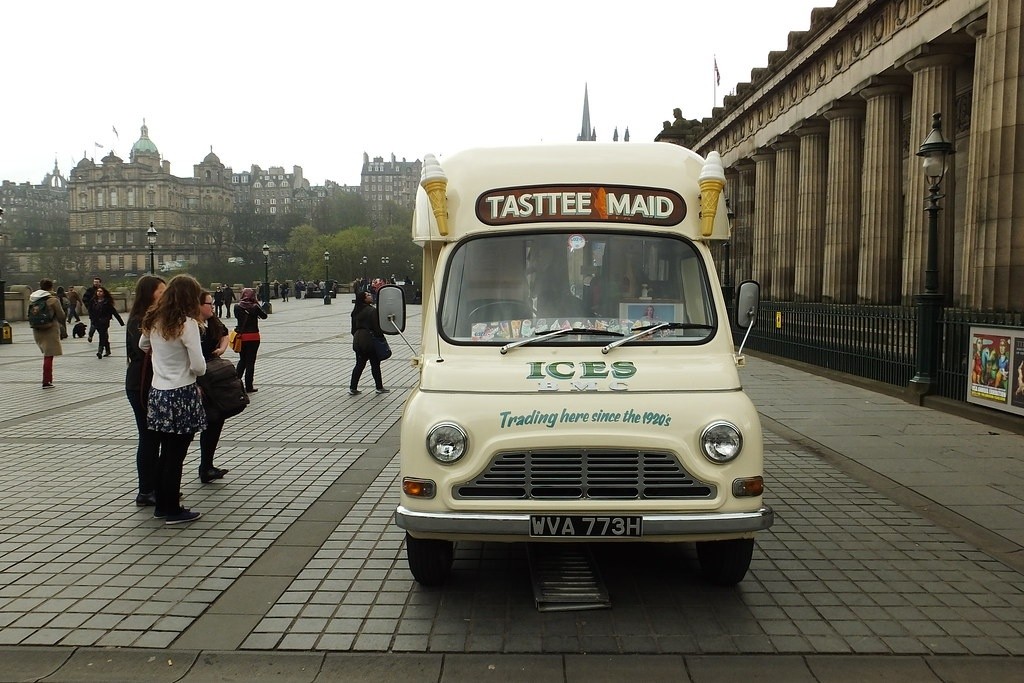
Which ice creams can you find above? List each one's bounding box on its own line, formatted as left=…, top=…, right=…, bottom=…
left=419, top=153, right=449, bottom=236
left=697, top=151, right=726, bottom=236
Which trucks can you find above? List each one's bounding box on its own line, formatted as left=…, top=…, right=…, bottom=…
left=377, top=141, right=775, bottom=590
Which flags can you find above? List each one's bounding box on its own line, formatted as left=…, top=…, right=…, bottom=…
left=715, top=59, right=720, bottom=86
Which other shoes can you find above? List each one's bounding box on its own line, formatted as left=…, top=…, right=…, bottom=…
left=200, top=467, right=228, bottom=483
left=103, top=352, right=111, bottom=356
left=376, top=387, right=390, bottom=394
left=136, top=492, right=156, bottom=507
left=61, top=334, right=68, bottom=340
left=41, top=383, right=56, bottom=388
left=348, top=388, right=363, bottom=395
left=75, top=319, right=80, bottom=322
left=248, top=387, right=258, bottom=392
left=97, top=352, right=102, bottom=359
left=226, top=316, right=230, bottom=318
left=67, top=320, right=71, bottom=324
left=88, top=335, right=92, bottom=343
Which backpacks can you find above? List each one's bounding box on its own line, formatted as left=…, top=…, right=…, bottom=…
left=28, top=296, right=55, bottom=329
left=197, top=353, right=251, bottom=420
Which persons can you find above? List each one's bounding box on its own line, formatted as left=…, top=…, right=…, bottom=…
left=88, top=287, right=125, bottom=359
left=27, top=279, right=66, bottom=389
left=82, top=278, right=102, bottom=343
left=196, top=292, right=230, bottom=483
left=125, top=276, right=166, bottom=506
left=347, top=291, right=393, bottom=395
left=404, top=276, right=412, bottom=285
left=65, top=286, right=83, bottom=323
left=234, top=288, right=268, bottom=392
left=214, top=287, right=224, bottom=318
left=353, top=274, right=397, bottom=306
left=662, top=108, right=686, bottom=130
left=55, top=286, right=69, bottom=340
left=137, top=273, right=207, bottom=524
left=222, top=284, right=236, bottom=318
left=256, top=279, right=306, bottom=303
left=307, top=279, right=338, bottom=298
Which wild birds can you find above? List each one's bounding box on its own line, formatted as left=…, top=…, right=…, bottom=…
left=94, top=142, right=103, bottom=148
left=113, top=126, right=118, bottom=137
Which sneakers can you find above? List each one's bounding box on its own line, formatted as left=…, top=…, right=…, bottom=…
left=153, top=505, right=191, bottom=519
left=165, top=511, right=201, bottom=524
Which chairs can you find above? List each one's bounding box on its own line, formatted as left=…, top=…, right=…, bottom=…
left=464, top=299, right=528, bottom=340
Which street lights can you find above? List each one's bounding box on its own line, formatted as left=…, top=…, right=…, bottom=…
left=909, top=112, right=958, bottom=396
left=261, top=240, right=273, bottom=314
left=716, top=196, right=735, bottom=323
left=324, top=249, right=332, bottom=305
left=362, top=255, right=371, bottom=285
left=144, top=221, right=159, bottom=273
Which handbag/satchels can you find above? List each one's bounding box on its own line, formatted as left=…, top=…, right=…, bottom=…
left=140, top=355, right=154, bottom=411
left=228, top=331, right=241, bottom=352
left=372, top=337, right=392, bottom=362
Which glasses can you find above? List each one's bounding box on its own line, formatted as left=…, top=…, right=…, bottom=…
left=204, top=300, right=216, bottom=307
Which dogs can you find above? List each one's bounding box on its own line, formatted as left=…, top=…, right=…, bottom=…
left=73, top=323, right=87, bottom=338
